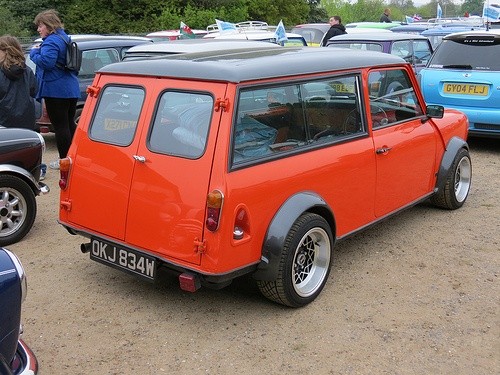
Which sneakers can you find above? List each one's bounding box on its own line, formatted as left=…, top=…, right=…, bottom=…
left=50, top=160, right=60, bottom=169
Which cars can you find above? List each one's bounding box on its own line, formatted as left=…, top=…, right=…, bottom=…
left=14, top=13, right=500, bottom=145
left=0, top=244, right=39, bottom=375
left=0, top=124, right=50, bottom=246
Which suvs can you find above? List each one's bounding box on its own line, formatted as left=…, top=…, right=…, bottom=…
left=57, top=45, right=474, bottom=307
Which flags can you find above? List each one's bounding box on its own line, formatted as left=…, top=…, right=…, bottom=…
left=482, top=3, right=500, bottom=22
left=413, top=13, right=422, bottom=21
left=180, top=21, right=196, bottom=40
left=437, top=3, right=442, bottom=19
left=215, top=19, right=238, bottom=33
left=274, top=19, right=288, bottom=42
left=405, top=16, right=414, bottom=24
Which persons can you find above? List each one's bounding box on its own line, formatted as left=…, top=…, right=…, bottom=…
left=380, top=8, right=392, bottom=23
left=0, top=36, right=38, bottom=130
left=30, top=9, right=81, bottom=169
left=323, top=16, right=347, bottom=46
left=463, top=10, right=469, bottom=17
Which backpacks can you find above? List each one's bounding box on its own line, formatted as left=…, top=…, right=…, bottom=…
left=44, top=32, right=83, bottom=73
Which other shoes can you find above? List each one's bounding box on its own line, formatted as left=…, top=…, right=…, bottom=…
left=40, top=169, right=45, bottom=180
